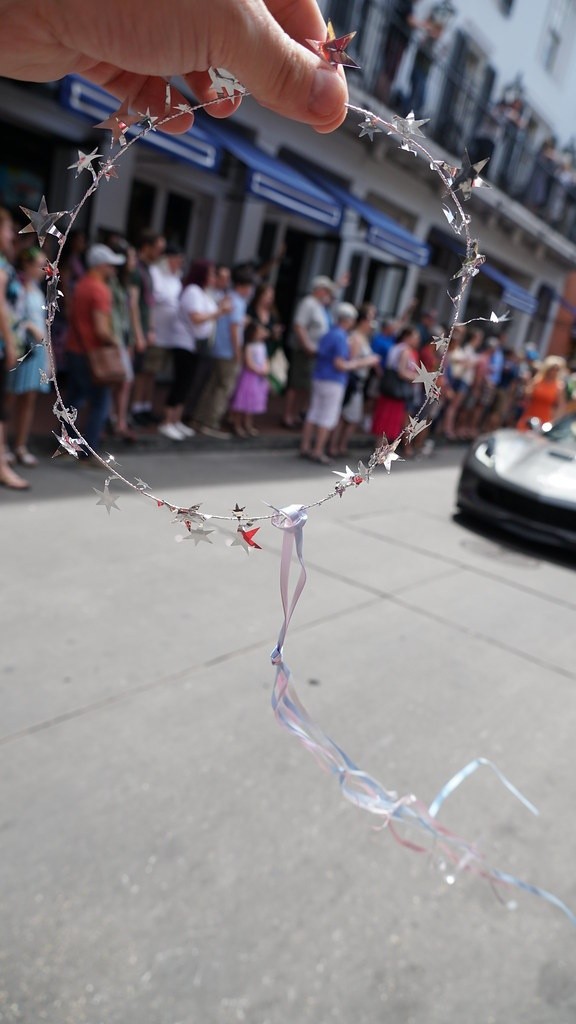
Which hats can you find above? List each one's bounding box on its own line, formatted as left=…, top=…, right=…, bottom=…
left=310, top=276, right=337, bottom=291
left=86, top=244, right=126, bottom=265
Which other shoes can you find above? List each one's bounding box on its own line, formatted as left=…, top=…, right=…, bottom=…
left=0, top=445, right=38, bottom=489
left=302, top=449, right=349, bottom=463
left=190, top=420, right=234, bottom=440
left=132, top=411, right=159, bottom=426
left=109, top=437, right=139, bottom=444
left=279, top=415, right=302, bottom=430
left=158, top=423, right=195, bottom=440
left=232, top=425, right=258, bottom=439
left=77, top=457, right=112, bottom=474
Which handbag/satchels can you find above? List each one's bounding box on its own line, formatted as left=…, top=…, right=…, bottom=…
left=380, top=370, right=414, bottom=400
left=85, top=345, right=126, bottom=385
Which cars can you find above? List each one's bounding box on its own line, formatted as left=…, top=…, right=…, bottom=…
left=456, top=410, right=576, bottom=551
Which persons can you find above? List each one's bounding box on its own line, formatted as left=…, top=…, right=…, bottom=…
left=0, top=1, right=576, bottom=490
